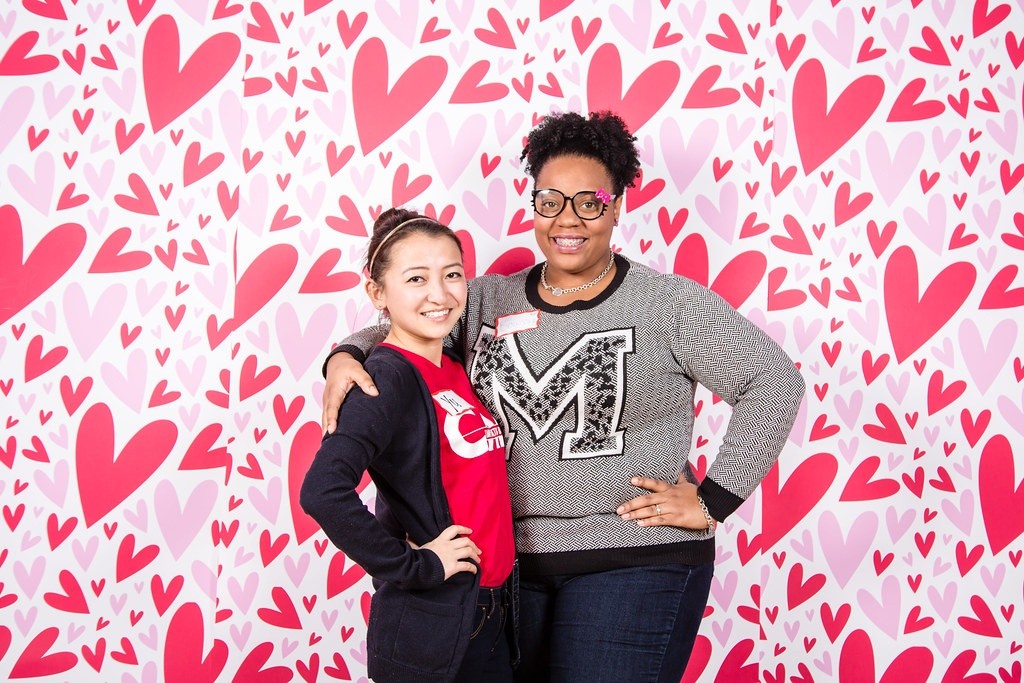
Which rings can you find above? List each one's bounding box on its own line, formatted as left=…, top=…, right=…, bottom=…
left=656, top=504, right=661, bottom=516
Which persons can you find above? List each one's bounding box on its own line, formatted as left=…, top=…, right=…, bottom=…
left=300, top=208, right=515, bottom=682
left=320, top=112, right=806, bottom=683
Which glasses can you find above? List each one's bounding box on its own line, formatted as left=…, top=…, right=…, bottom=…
left=532, top=187, right=617, bottom=221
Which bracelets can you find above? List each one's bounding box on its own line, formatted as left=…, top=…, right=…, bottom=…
left=697, top=492, right=715, bottom=534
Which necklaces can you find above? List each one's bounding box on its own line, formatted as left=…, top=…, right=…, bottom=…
left=541, top=247, right=615, bottom=296
